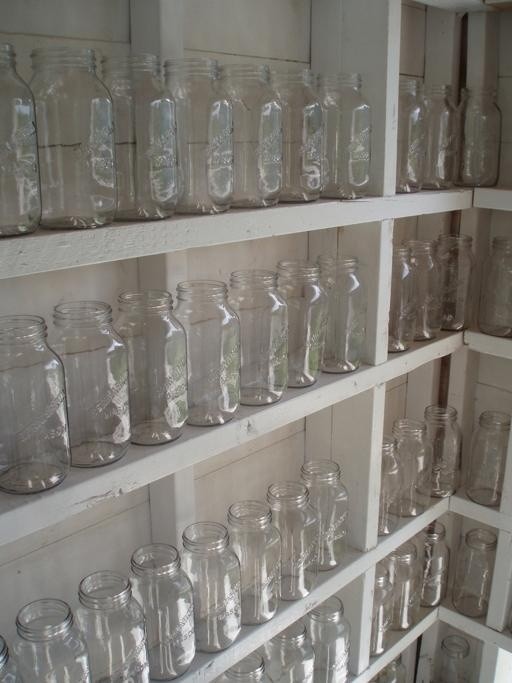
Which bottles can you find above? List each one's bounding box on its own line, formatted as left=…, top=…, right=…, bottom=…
left=2, top=46, right=511, bottom=682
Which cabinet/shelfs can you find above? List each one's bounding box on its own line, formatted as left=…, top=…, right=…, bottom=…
left=1, top=1, right=512, bottom=683
